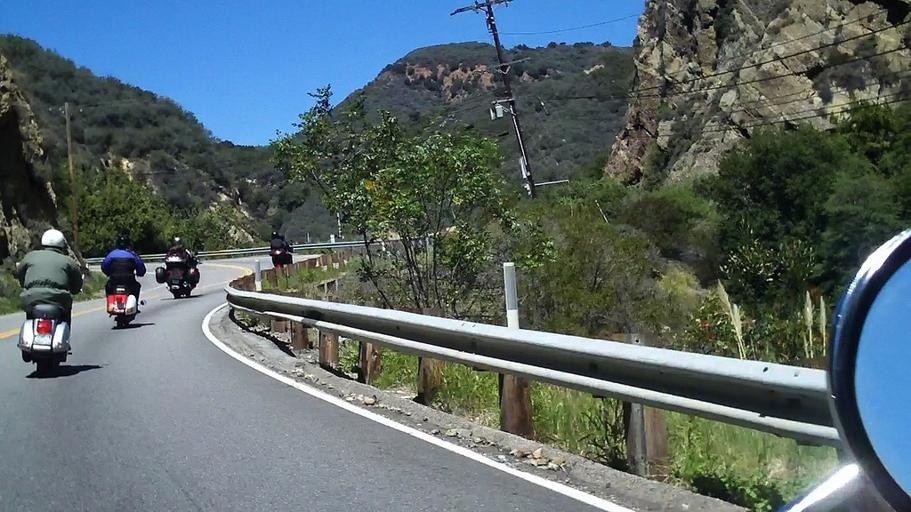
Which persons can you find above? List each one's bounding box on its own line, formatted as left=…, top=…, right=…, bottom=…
left=271, top=231, right=293, bottom=267
left=165, top=236, right=198, bottom=267
left=12, top=227, right=85, bottom=322
left=279, top=233, right=293, bottom=253
left=101, top=233, right=147, bottom=300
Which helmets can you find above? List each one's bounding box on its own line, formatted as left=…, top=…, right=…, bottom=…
left=271, top=231, right=279, bottom=238
left=116, top=237, right=129, bottom=248
left=172, top=237, right=182, bottom=245
left=41, top=229, right=66, bottom=249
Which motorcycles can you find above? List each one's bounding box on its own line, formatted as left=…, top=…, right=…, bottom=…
left=12, top=269, right=72, bottom=377
left=269, top=240, right=293, bottom=269
left=106, top=285, right=138, bottom=327
left=156, top=250, right=201, bottom=299
